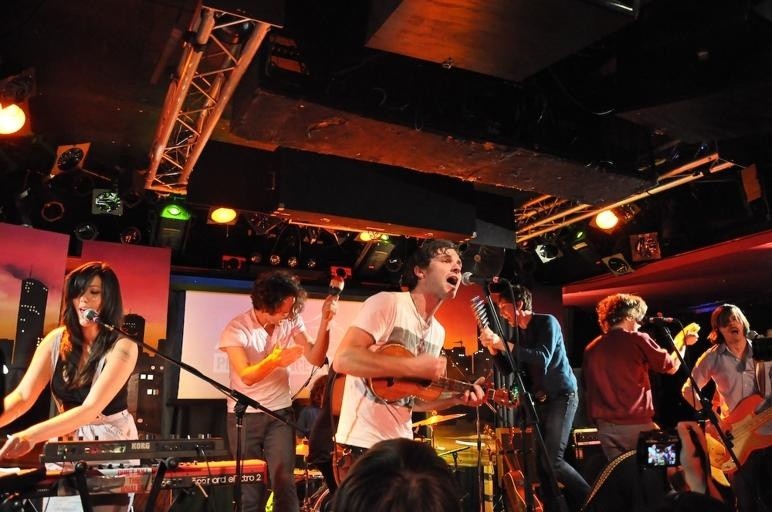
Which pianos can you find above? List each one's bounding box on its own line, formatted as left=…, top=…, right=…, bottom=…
left=0, top=435, right=269, bottom=493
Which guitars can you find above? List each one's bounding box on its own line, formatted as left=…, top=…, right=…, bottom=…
left=366, top=343, right=519, bottom=409
left=703, top=394, right=772, bottom=470
left=484, top=424, right=544, bottom=511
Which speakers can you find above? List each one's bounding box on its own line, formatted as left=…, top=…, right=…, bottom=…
left=141, top=203, right=189, bottom=257
left=344, top=237, right=393, bottom=276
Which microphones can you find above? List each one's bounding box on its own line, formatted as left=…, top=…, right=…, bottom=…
left=462, top=272, right=508, bottom=285
left=748, top=331, right=758, bottom=339
left=322, top=276, right=345, bottom=320
left=649, top=317, right=679, bottom=324
left=83, top=309, right=113, bottom=332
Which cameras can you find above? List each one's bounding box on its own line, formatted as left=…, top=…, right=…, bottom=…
left=638, top=431, right=680, bottom=466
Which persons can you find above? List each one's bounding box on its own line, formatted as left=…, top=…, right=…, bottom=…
left=218, top=271, right=340, bottom=512
left=309, top=361, right=346, bottom=494
left=165, top=324, right=191, bottom=440
left=581, top=293, right=700, bottom=463
left=331, top=238, right=486, bottom=486
left=480, top=285, right=593, bottom=512
left=296, top=374, right=328, bottom=444
left=681, top=303, right=772, bottom=512
left=646, top=420, right=734, bottom=511
left=0, top=261, right=140, bottom=512
left=329, top=438, right=463, bottom=512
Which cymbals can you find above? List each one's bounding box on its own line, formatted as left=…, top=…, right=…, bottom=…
left=409, top=414, right=465, bottom=426
